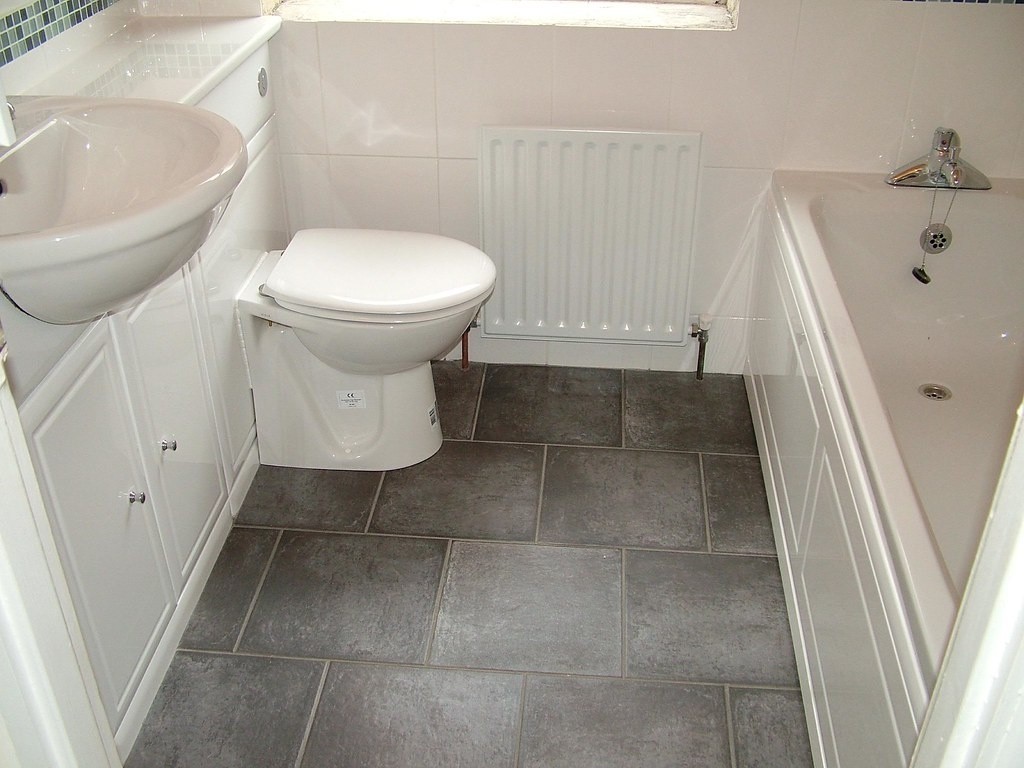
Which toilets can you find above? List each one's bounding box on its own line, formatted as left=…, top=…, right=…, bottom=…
left=236, top=226, right=496, bottom=470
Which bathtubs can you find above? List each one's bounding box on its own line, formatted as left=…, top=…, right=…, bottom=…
left=747, top=166, right=1024, bottom=768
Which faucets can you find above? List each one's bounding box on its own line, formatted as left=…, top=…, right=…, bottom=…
left=886, top=128, right=993, bottom=189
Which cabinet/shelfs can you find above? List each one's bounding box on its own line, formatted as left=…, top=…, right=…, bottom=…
left=14, top=111, right=292, bottom=768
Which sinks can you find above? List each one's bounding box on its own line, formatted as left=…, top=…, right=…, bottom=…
left=0, top=92, right=249, bottom=324
